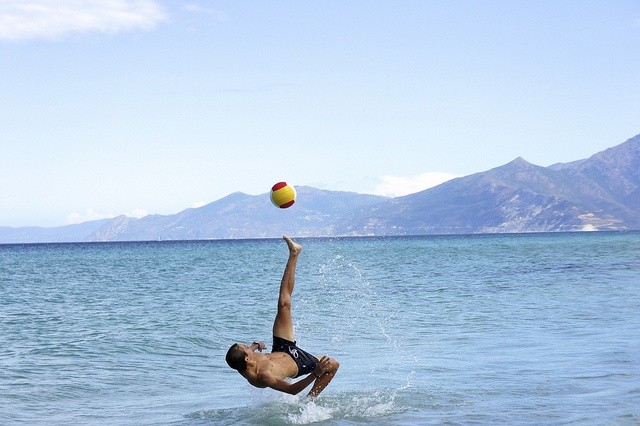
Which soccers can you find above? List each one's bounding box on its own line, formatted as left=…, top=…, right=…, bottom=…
left=270, top=182, right=296, bottom=208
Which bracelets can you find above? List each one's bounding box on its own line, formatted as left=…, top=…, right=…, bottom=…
left=252, top=342, right=260, bottom=349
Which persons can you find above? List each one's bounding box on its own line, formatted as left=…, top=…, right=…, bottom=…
left=225, top=234, right=340, bottom=399
